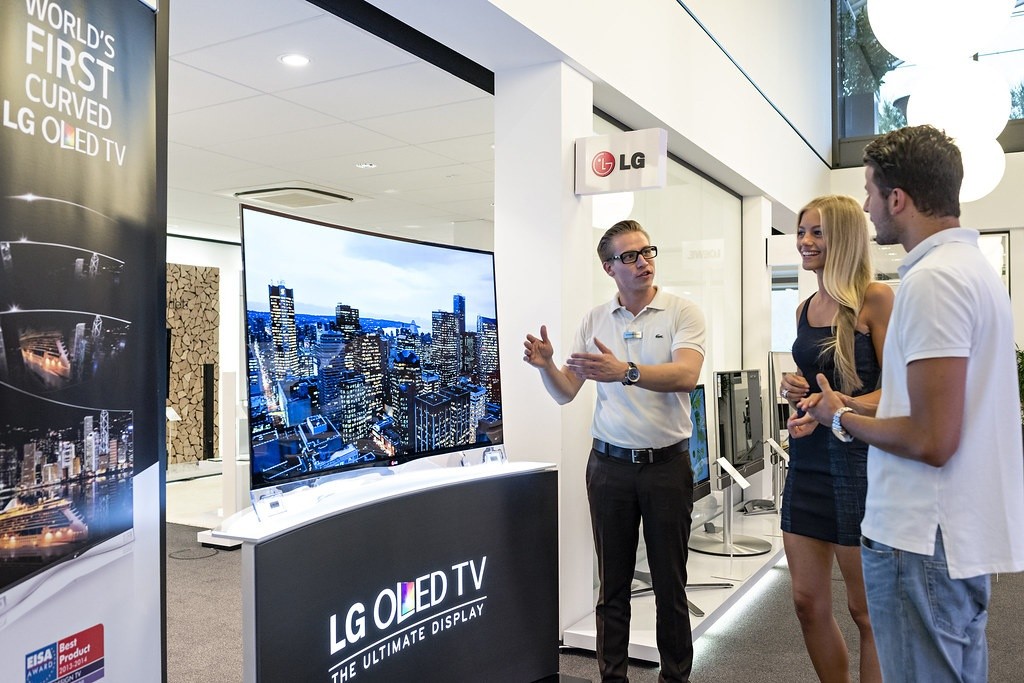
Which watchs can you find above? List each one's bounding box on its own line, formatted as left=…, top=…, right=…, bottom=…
left=831, top=407, right=858, bottom=443
left=622, top=362, right=642, bottom=386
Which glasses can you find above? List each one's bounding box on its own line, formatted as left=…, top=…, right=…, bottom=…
left=606, top=246, right=658, bottom=264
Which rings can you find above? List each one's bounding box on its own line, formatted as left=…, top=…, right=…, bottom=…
left=781, top=389, right=790, bottom=400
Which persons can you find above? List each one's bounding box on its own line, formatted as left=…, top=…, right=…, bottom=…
left=801, top=124, right=1024, bottom=683
left=522, top=220, right=706, bottom=683
left=780, top=195, right=896, bottom=683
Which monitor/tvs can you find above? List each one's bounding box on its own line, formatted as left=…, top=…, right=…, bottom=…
left=239, top=202, right=505, bottom=491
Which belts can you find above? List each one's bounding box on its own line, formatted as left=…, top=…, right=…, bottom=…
left=592, top=438, right=689, bottom=464
